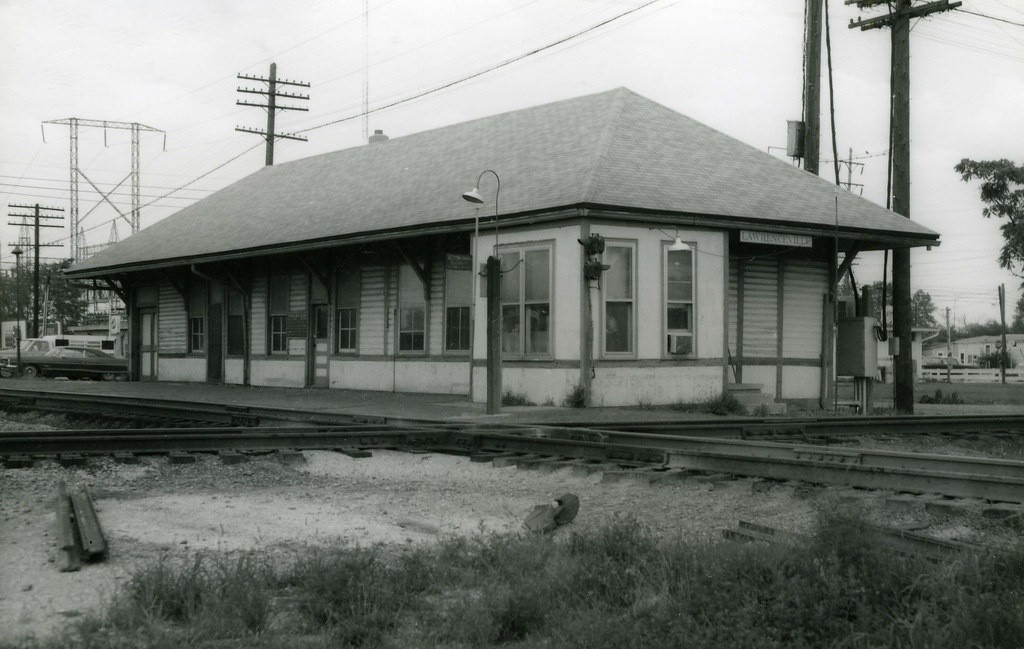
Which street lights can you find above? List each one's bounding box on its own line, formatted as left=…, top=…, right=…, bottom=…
left=11, top=247, right=23, bottom=376
left=462, top=170, right=502, bottom=414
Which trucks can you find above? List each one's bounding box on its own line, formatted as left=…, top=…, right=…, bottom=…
left=0, top=334, right=115, bottom=379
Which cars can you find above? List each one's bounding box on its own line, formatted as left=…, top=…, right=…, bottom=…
left=921, top=356, right=961, bottom=376
left=17, top=346, right=127, bottom=381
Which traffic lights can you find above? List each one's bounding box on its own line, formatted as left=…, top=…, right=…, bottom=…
left=579, top=235, right=605, bottom=255
